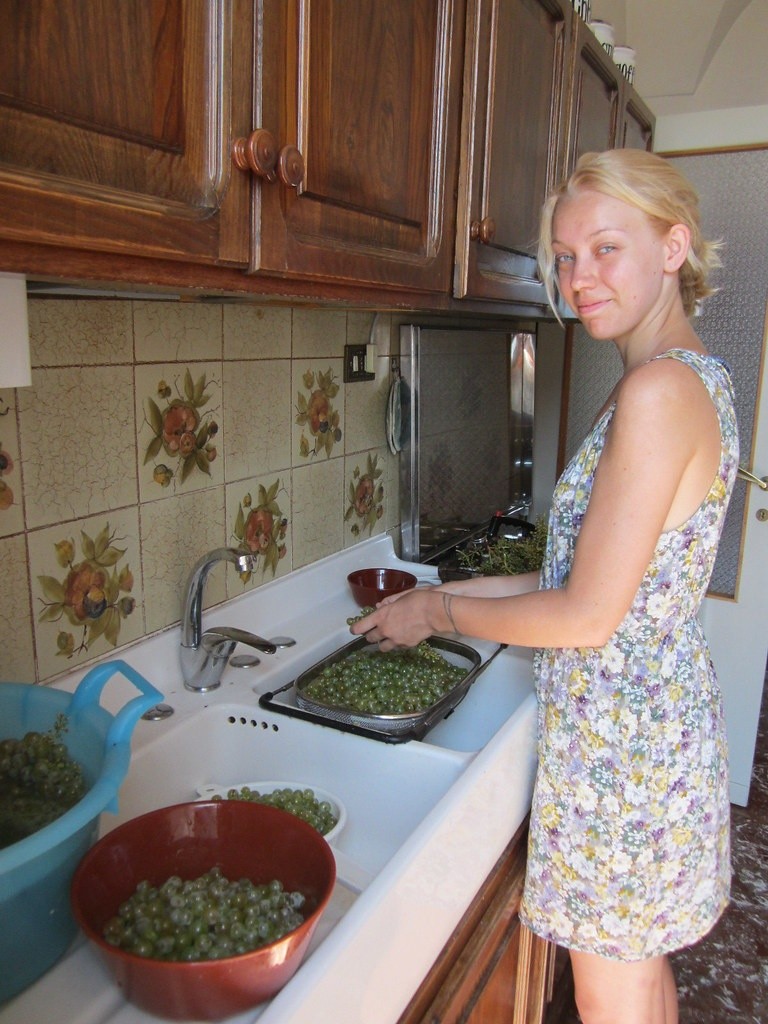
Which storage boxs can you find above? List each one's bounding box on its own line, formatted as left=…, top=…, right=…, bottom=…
left=69, top=791, right=330, bottom=1023
left=293, top=626, right=489, bottom=742
left=0, top=654, right=161, bottom=1000
left=204, top=778, right=352, bottom=842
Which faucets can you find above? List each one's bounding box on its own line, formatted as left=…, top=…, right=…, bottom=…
left=178, top=546, right=277, bottom=692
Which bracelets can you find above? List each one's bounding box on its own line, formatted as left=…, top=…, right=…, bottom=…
left=442, top=593, right=462, bottom=636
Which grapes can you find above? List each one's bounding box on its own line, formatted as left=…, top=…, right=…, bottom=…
left=0, top=513, right=547, bottom=962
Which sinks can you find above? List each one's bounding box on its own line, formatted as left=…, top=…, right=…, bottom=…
left=102, top=705, right=467, bottom=948
left=251, top=602, right=538, bottom=756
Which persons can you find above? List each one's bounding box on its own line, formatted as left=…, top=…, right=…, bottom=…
left=344, top=147, right=745, bottom=1024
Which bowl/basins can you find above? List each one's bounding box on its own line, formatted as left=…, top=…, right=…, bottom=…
left=347, top=568, right=417, bottom=609
left=70, top=799, right=333, bottom=1023
left=192, top=780, right=348, bottom=846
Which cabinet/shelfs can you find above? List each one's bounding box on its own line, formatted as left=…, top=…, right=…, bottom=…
left=1, top=1, right=663, bottom=332
